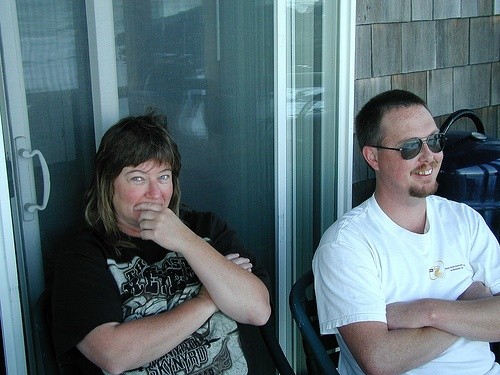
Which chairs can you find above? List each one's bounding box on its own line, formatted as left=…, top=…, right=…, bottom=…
left=289, top=271, right=496, bottom=375
left=32, top=287, right=295, bottom=375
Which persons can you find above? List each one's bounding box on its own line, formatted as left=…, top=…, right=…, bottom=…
left=311, top=88, right=500, bottom=375
left=46, top=115, right=271, bottom=375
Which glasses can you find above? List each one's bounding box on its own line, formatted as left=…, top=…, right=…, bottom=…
left=370, top=133, right=447, bottom=160
